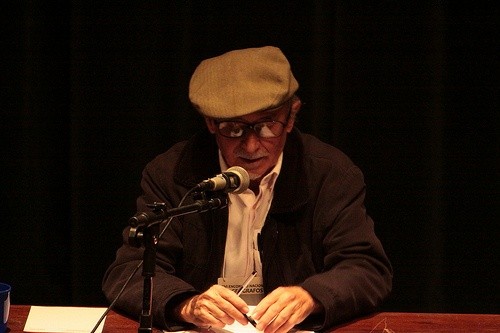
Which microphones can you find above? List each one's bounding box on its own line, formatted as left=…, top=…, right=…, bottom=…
left=196, top=165, right=250, bottom=195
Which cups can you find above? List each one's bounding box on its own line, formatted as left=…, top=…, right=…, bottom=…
left=0, top=282, right=12, bottom=333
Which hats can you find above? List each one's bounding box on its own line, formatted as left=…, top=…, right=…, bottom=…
left=188, top=46, right=299, bottom=119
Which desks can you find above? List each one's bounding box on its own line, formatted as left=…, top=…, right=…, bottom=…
left=7, top=305, right=500, bottom=333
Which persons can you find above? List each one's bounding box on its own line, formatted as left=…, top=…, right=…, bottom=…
left=101, top=45, right=393, bottom=333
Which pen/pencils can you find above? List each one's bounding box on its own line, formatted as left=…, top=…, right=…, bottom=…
left=243, top=314, right=257, bottom=327
left=236, top=270, right=258, bottom=296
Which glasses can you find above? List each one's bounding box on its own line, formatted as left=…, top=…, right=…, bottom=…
left=213, top=111, right=291, bottom=138
